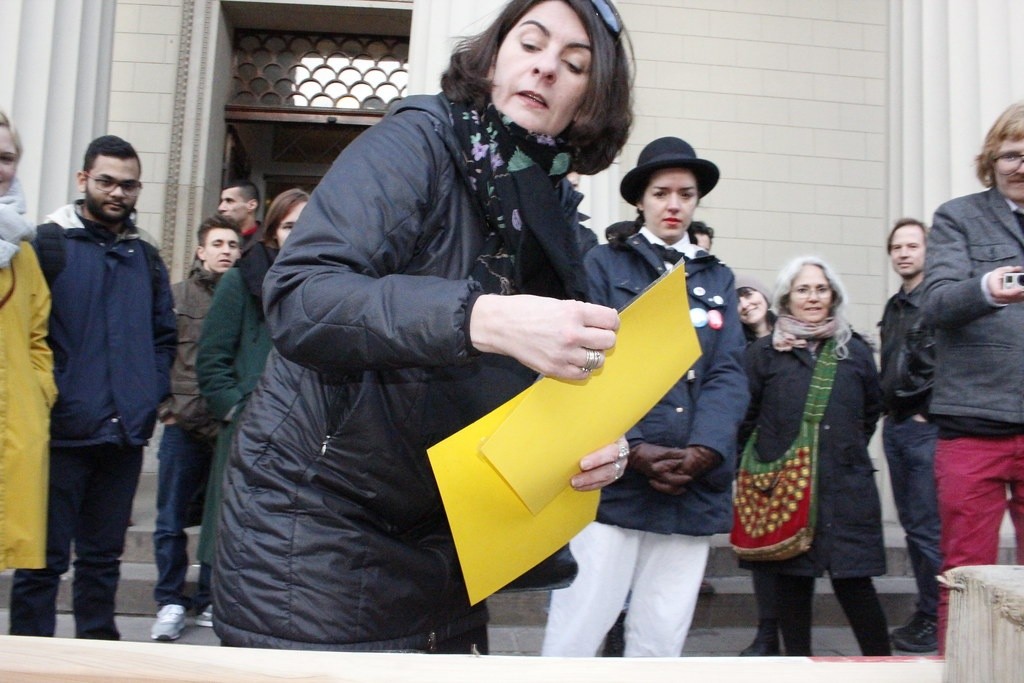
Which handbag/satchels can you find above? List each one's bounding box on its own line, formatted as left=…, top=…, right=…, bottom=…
left=731, top=434, right=817, bottom=564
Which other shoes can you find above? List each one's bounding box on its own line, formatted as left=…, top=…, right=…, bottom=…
left=700, top=580, right=714, bottom=594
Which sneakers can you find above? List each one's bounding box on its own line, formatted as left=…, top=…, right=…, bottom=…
left=151, top=604, right=190, bottom=640
left=195, top=605, right=214, bottom=627
left=890, top=609, right=940, bottom=651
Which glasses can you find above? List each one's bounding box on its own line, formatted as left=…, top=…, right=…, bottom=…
left=86, top=174, right=141, bottom=197
left=793, top=286, right=830, bottom=300
left=993, top=153, right=1024, bottom=175
left=592, top=0, right=620, bottom=35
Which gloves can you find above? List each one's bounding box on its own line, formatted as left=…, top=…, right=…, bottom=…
left=650, top=446, right=717, bottom=496
left=629, top=442, right=687, bottom=493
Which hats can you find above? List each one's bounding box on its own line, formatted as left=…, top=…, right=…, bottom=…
left=735, top=282, right=773, bottom=309
left=621, top=138, right=719, bottom=206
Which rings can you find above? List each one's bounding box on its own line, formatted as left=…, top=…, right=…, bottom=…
left=583, top=348, right=600, bottom=372
left=619, top=444, right=629, bottom=459
left=615, top=463, right=620, bottom=479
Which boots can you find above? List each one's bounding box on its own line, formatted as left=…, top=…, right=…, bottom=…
left=739, top=573, right=781, bottom=657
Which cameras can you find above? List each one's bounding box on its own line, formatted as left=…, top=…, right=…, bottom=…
left=1002, top=273, right=1024, bottom=289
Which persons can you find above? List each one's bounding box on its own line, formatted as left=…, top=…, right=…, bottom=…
left=217, top=178, right=261, bottom=243
left=151, top=213, right=244, bottom=641
left=542, top=137, right=750, bottom=658
left=876, top=219, right=941, bottom=652
left=195, top=189, right=306, bottom=567
left=733, top=275, right=780, bottom=655
left=738, top=260, right=892, bottom=655
left=690, top=222, right=716, bottom=593
left=10, top=136, right=178, bottom=638
left=0, top=111, right=59, bottom=571
left=923, top=97, right=1024, bottom=656
left=211, top=0, right=638, bottom=657
